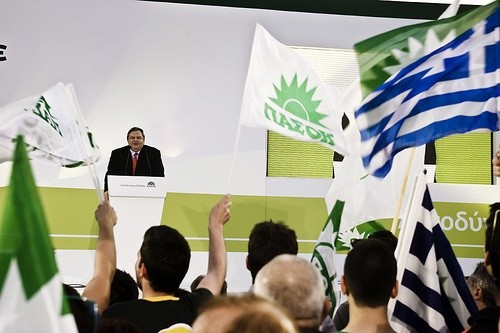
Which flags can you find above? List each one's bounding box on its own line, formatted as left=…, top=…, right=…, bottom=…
left=310, top=200, right=398, bottom=323
left=352, top=0, right=500, bottom=102
left=354, top=11, right=500, bottom=176
left=0, top=135, right=79, bottom=332
left=0, top=81, right=102, bottom=170
left=387, top=184, right=480, bottom=333
left=239, top=22, right=348, bottom=156
left=333, top=112, right=437, bottom=184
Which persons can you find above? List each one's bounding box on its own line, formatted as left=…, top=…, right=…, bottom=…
left=103, top=127, right=164, bottom=201
left=334, top=239, right=398, bottom=332
left=61, top=202, right=118, bottom=333
left=246, top=221, right=299, bottom=287
left=191, top=274, right=228, bottom=297
left=109, top=268, right=139, bottom=306
left=332, top=237, right=369, bottom=331
left=252, top=254, right=331, bottom=332
left=460, top=202, right=500, bottom=333
left=192, top=295, right=298, bottom=333
left=370, top=230, right=398, bottom=252
left=465, top=261, right=496, bottom=310
left=98, top=192, right=233, bottom=333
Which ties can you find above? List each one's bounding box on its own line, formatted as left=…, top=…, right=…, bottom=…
left=132, top=153, right=137, bottom=176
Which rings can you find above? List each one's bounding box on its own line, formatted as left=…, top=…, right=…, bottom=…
left=105, top=198, right=109, bottom=200
